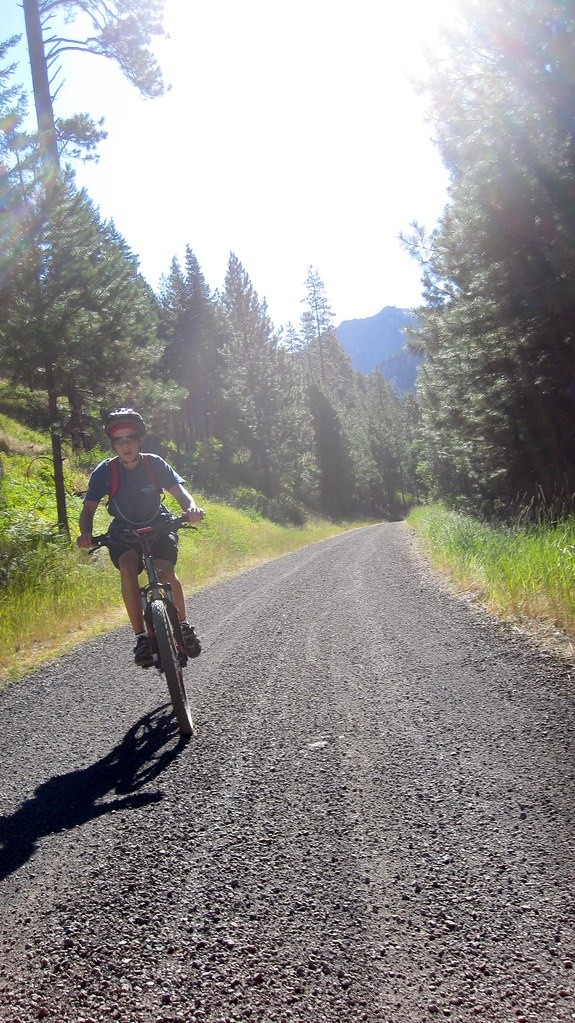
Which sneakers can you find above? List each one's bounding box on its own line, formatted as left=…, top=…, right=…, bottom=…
left=133, top=636, right=153, bottom=666
left=180, top=624, right=201, bottom=658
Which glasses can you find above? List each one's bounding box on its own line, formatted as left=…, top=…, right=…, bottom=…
left=112, top=433, right=140, bottom=445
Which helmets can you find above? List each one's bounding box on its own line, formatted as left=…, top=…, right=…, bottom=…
left=104, top=407, right=147, bottom=437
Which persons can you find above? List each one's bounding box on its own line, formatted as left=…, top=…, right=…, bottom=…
left=76, top=407, right=203, bottom=666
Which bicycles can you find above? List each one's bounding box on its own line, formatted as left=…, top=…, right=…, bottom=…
left=85, top=515, right=198, bottom=737
